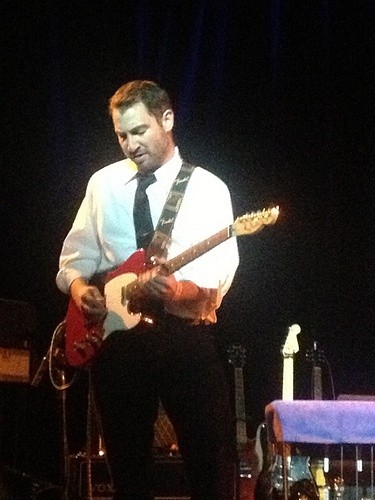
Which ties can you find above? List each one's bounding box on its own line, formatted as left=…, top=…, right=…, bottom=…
left=133, top=175, right=157, bottom=252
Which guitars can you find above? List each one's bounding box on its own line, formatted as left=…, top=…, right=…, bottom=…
left=304, top=341, right=343, bottom=500
left=67, top=206, right=279, bottom=364
left=223, top=342, right=259, bottom=500
left=255, top=324, right=320, bottom=500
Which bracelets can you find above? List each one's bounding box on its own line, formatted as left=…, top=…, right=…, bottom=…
left=171, top=281, right=183, bottom=303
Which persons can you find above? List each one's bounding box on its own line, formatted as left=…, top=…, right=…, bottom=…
left=56, top=80, right=245, bottom=500
